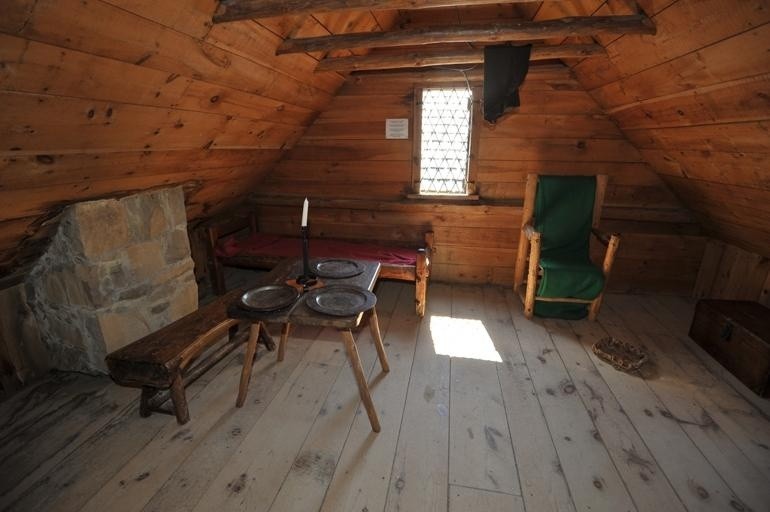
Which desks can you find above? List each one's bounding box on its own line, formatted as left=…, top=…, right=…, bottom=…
left=228, top=258, right=390, bottom=432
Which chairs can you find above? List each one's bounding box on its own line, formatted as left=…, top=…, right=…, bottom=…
left=515, top=173, right=621, bottom=320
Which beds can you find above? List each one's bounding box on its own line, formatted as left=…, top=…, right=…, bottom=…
left=207, top=209, right=434, bottom=318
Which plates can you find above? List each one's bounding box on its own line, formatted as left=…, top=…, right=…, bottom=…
left=314, top=257, right=367, bottom=279
left=305, top=284, right=377, bottom=316
left=240, top=283, right=300, bottom=311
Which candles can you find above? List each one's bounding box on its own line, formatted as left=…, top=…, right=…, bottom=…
left=302, top=196, right=309, bottom=227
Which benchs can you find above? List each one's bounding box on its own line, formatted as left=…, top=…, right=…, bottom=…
left=104, top=277, right=279, bottom=425
